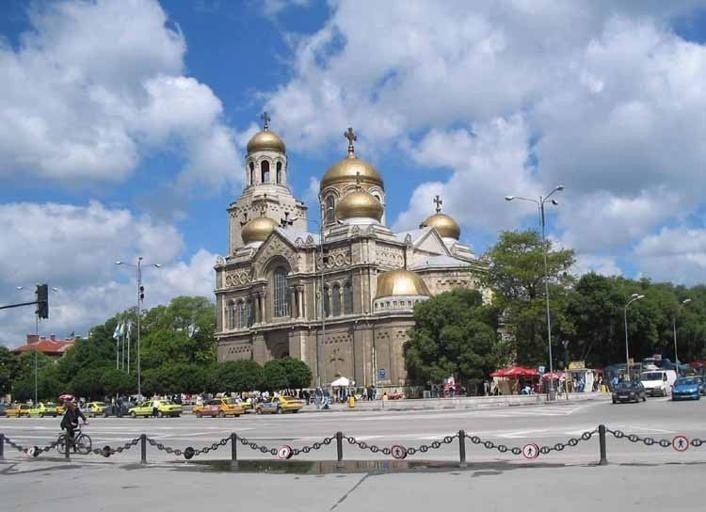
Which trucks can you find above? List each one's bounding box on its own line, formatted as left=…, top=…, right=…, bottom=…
left=640, top=369, right=677, bottom=397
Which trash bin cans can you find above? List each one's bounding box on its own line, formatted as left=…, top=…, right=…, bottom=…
left=431, top=384, right=436, bottom=397
left=350, top=398, right=355, bottom=408
left=600, top=386, right=606, bottom=393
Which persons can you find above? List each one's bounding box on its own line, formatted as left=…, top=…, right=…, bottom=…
left=381, top=391, right=389, bottom=401
left=404, top=369, right=666, bottom=400
left=24, top=398, right=34, bottom=416
left=59, top=400, right=89, bottom=454
left=390, top=389, right=398, bottom=396
left=101, top=384, right=379, bottom=418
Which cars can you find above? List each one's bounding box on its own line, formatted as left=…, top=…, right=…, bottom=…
left=0, top=401, right=112, bottom=418
left=127, top=399, right=182, bottom=418
left=255, top=395, right=303, bottom=414
left=613, top=380, right=647, bottom=403
left=192, top=397, right=245, bottom=417
left=671, top=376, right=706, bottom=401
left=233, top=397, right=252, bottom=409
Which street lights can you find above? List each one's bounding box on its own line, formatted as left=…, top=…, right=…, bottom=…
left=673, top=298, right=692, bottom=373
left=115, top=256, right=160, bottom=405
left=624, top=293, right=647, bottom=381
left=505, top=185, right=566, bottom=402
left=17, top=285, right=58, bottom=405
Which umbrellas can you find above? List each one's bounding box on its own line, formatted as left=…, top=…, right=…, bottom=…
left=328, top=376, right=355, bottom=398
left=56, top=393, right=75, bottom=402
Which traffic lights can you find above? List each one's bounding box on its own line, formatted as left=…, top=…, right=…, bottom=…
left=139, top=284, right=144, bottom=302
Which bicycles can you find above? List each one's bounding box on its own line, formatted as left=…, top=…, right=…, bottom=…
left=56, top=423, right=91, bottom=454
